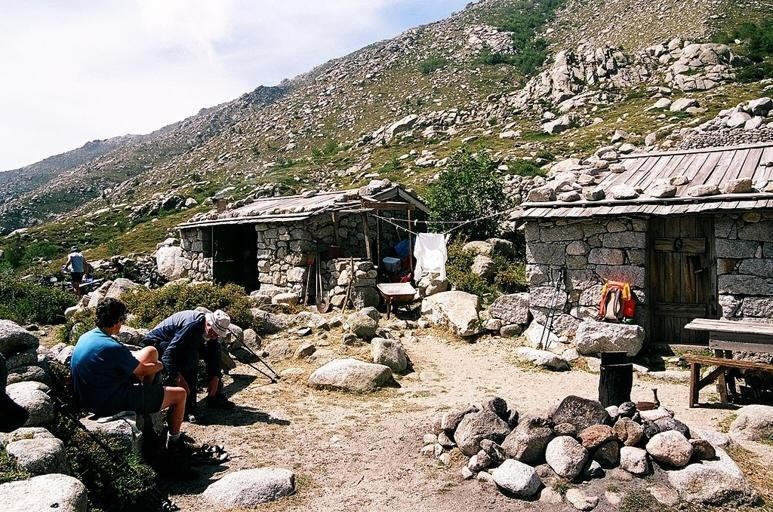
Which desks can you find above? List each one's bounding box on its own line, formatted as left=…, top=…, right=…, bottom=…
left=684, top=318, right=773, bottom=404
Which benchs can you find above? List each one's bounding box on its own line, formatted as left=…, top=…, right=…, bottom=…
left=679, top=353, right=773, bottom=409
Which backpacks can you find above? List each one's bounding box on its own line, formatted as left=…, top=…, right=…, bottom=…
left=596, top=280, right=636, bottom=323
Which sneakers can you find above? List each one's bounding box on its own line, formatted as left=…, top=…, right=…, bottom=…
left=168, top=437, right=201, bottom=455
left=205, top=391, right=235, bottom=410
left=186, top=410, right=209, bottom=423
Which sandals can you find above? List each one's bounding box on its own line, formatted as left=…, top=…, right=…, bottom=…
left=198, top=444, right=224, bottom=455
left=200, top=452, right=228, bottom=465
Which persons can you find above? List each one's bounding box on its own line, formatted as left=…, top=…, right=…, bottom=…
left=138, top=308, right=235, bottom=422
left=64, top=246, right=88, bottom=296
left=71, top=297, right=195, bottom=456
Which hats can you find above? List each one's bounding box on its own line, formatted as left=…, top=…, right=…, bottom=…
left=205, top=309, right=231, bottom=337
